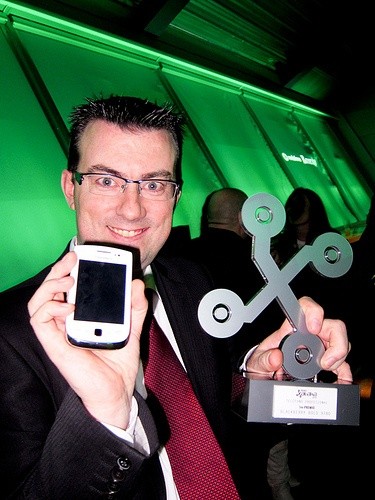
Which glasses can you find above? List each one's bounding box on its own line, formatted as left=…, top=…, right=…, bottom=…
left=72, top=170, right=180, bottom=201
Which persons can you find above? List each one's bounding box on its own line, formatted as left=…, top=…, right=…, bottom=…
left=264, top=186, right=349, bottom=500
left=0, top=91, right=353, bottom=500
left=305, top=195, right=375, bottom=500
left=155, top=187, right=268, bottom=500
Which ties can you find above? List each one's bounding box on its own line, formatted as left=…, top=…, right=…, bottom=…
left=139, top=289, right=240, bottom=500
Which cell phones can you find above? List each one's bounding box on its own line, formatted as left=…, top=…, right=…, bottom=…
left=65, top=244, right=132, bottom=350
